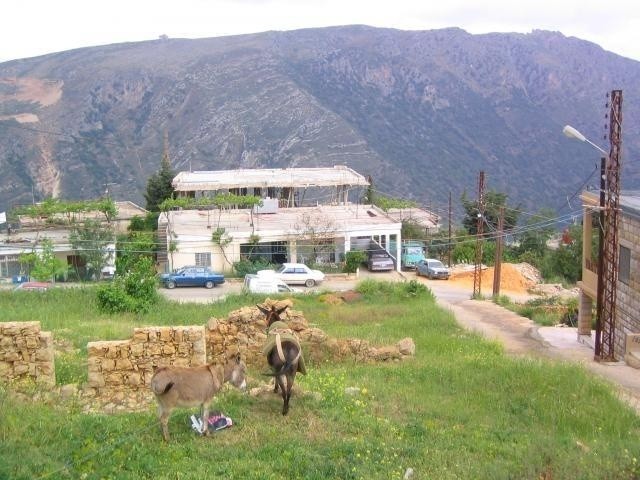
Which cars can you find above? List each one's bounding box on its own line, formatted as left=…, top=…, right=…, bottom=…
left=159, top=265, right=225, bottom=289
left=414, top=259, right=450, bottom=280
left=276, top=262, right=325, bottom=288
left=364, top=248, right=394, bottom=271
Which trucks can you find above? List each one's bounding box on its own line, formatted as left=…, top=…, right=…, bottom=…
left=389, top=239, right=426, bottom=271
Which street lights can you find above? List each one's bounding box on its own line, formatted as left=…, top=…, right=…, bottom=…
left=562, top=125, right=612, bottom=360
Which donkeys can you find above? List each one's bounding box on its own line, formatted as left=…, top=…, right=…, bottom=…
left=151, top=340, right=247, bottom=441
left=257, top=305, right=301, bottom=416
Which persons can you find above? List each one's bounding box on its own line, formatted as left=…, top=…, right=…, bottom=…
left=562, top=227, right=573, bottom=245
